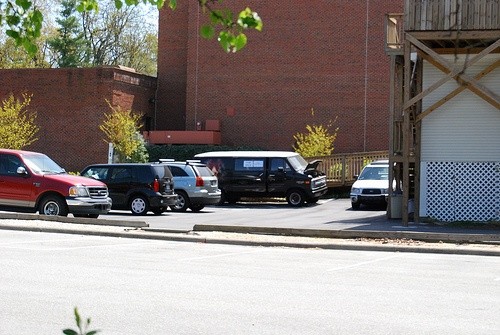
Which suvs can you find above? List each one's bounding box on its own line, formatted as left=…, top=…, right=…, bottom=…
left=149, top=157, right=223, bottom=212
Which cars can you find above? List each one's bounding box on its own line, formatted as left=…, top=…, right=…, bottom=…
left=349, top=160, right=403, bottom=210
left=0, top=148, right=112, bottom=218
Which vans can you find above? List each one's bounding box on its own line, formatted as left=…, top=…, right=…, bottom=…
left=195, top=149, right=330, bottom=208
left=76, top=162, right=178, bottom=216
